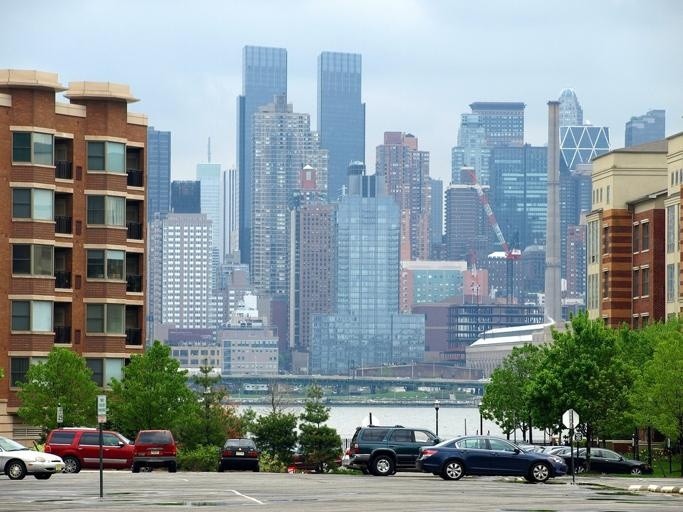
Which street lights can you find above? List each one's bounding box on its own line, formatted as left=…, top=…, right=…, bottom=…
left=477, top=402, right=484, bottom=435
left=202, top=387, right=213, bottom=442
left=434, top=399, right=440, bottom=436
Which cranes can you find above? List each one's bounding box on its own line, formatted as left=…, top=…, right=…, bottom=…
left=470, top=170, right=521, bottom=305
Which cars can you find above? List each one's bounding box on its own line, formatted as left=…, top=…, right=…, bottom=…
left=45, top=428, right=153, bottom=473
left=218, top=438, right=260, bottom=472
left=133, top=429, right=176, bottom=472
left=287, top=447, right=342, bottom=474
left=0, top=436, right=65, bottom=480
